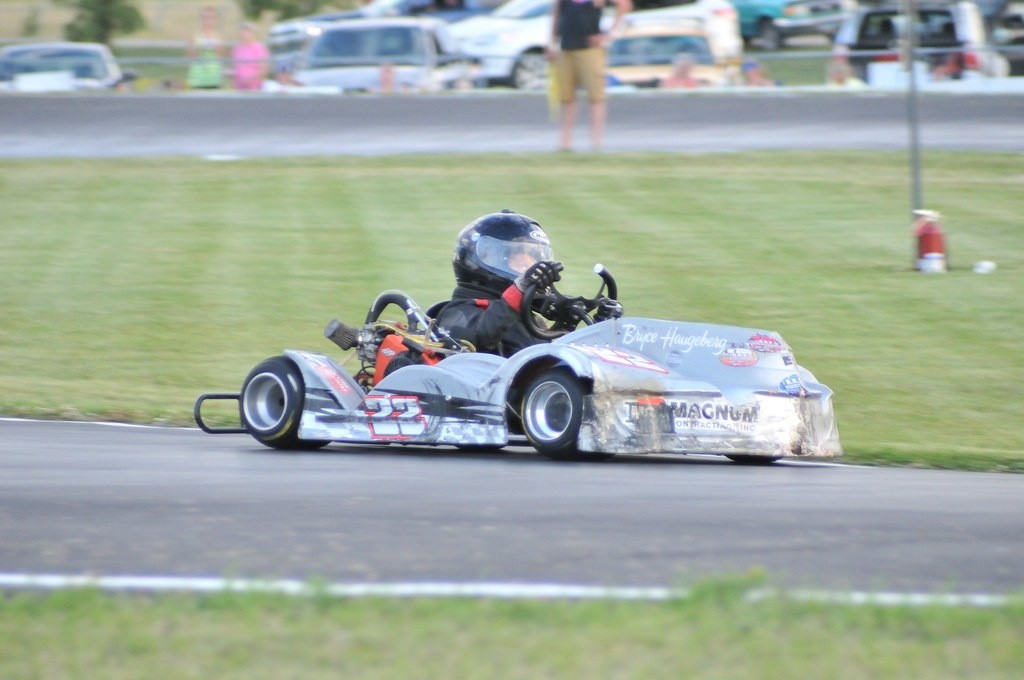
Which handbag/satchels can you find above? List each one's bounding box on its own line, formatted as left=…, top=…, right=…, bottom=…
left=546, top=62, right=559, bottom=110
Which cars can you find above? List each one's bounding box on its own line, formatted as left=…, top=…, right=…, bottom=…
left=598, top=18, right=729, bottom=91
left=0, top=43, right=127, bottom=98
left=446, top=0, right=619, bottom=91
left=832, top=7, right=972, bottom=84
left=293, top=17, right=459, bottom=97
left=724, top=1, right=843, bottom=49
left=963, top=4, right=1024, bottom=78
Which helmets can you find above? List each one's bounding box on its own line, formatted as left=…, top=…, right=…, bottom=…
left=452, top=209, right=552, bottom=297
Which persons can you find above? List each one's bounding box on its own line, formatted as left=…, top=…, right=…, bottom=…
left=431, top=209, right=625, bottom=360
left=183, top=6, right=419, bottom=93
left=545, top=0, right=988, bottom=156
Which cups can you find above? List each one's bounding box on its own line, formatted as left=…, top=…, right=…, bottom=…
left=921, top=252, right=945, bottom=273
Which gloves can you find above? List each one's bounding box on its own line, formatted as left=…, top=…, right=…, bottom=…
left=515, top=261, right=561, bottom=289
left=592, top=297, right=624, bottom=323
